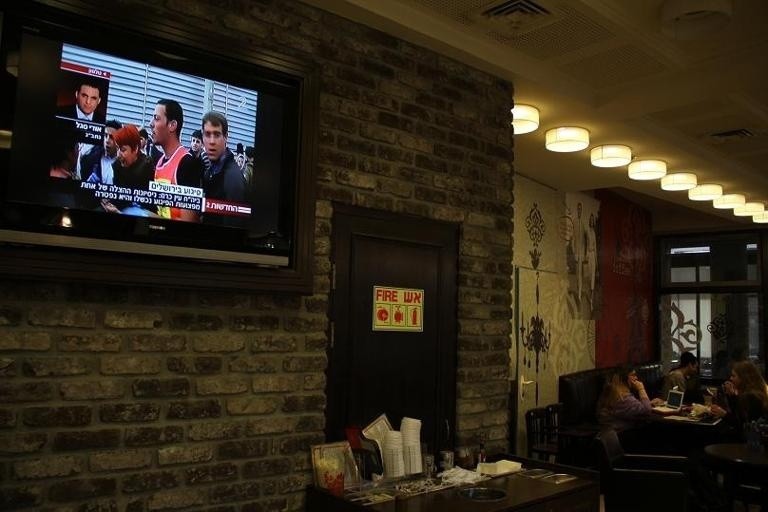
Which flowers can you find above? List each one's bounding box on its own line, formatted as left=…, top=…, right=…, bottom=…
left=743, top=418, right=768, bottom=442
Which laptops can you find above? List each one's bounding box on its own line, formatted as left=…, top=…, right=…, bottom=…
left=654, top=389, right=685, bottom=416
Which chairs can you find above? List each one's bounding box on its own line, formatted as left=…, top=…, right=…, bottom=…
left=525, top=403, right=565, bottom=460
left=593, top=425, right=687, bottom=512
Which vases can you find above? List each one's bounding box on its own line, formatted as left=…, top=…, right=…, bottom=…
left=752, top=440, right=761, bottom=450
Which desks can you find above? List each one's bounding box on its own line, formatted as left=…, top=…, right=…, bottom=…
left=309, top=454, right=594, bottom=512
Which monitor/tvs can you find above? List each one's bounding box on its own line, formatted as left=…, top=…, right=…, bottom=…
left=1, top=0, right=302, bottom=268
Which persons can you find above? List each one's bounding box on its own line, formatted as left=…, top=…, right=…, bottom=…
left=662, top=351, right=702, bottom=405
left=48, top=78, right=254, bottom=231
left=596, top=363, right=662, bottom=452
left=706, top=361, right=767, bottom=445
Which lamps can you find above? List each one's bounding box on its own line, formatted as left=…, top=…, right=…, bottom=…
left=511, top=103, right=768, bottom=225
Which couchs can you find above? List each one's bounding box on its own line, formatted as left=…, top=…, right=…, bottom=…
left=556, top=361, right=663, bottom=472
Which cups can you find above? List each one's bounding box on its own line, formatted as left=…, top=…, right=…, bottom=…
left=709, top=387, right=718, bottom=397
left=704, top=395, right=712, bottom=408
left=381, top=417, right=425, bottom=479
